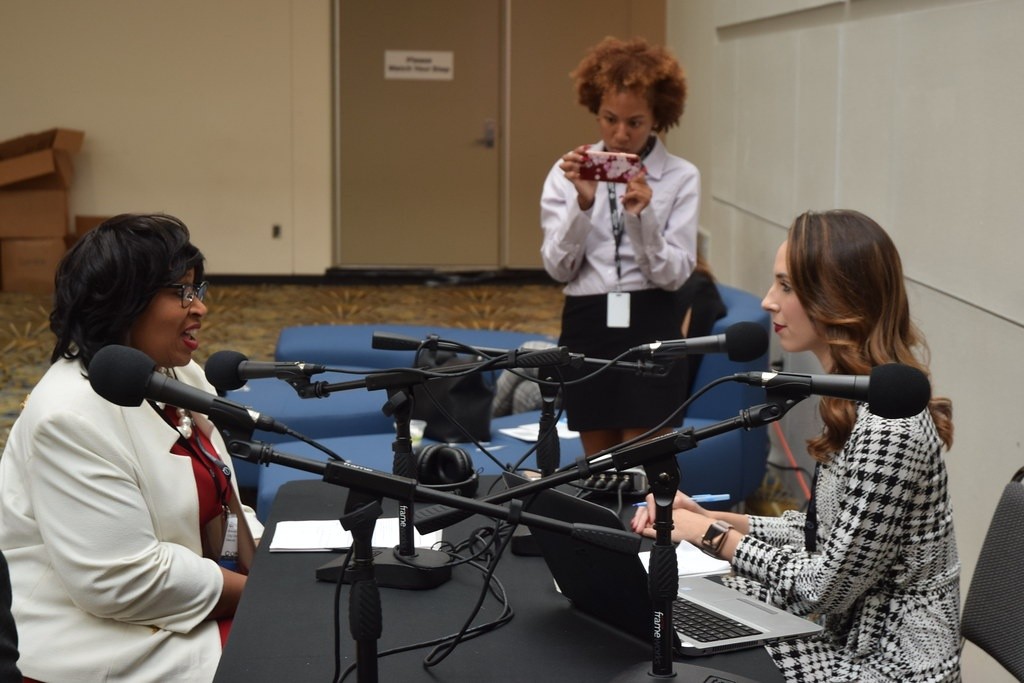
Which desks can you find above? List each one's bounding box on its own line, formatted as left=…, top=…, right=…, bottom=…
left=214, top=481, right=785, bottom=683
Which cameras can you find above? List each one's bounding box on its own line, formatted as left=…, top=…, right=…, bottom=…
left=575, top=150, right=641, bottom=183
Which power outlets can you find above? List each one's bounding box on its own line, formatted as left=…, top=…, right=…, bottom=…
left=272, top=225, right=281, bottom=238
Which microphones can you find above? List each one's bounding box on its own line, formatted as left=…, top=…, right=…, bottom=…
left=733, top=363, right=932, bottom=419
left=87, top=345, right=289, bottom=435
left=204, top=350, right=326, bottom=390
left=636, top=321, right=770, bottom=363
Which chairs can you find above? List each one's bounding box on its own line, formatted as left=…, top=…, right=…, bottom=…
left=958, top=466, right=1024, bottom=683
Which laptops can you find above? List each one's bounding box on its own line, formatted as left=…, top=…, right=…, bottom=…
left=499, top=471, right=828, bottom=657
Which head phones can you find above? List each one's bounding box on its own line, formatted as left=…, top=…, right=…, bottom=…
left=414, top=441, right=481, bottom=494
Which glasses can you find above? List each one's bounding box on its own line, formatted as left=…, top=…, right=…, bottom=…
left=162, top=281, right=209, bottom=308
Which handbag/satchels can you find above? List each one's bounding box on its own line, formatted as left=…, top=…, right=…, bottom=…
left=417, top=349, right=491, bottom=443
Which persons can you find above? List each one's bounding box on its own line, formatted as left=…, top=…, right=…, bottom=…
left=0, top=210, right=265, bottom=683
left=536, top=32, right=702, bottom=468
left=631, top=208, right=963, bottom=683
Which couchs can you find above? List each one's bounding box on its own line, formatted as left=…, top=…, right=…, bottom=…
left=216, top=365, right=393, bottom=498
left=256, top=278, right=772, bottom=530
left=274, top=327, right=560, bottom=384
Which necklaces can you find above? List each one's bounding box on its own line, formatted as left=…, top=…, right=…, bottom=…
left=154, top=366, right=192, bottom=439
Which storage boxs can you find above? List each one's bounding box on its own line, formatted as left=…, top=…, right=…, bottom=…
left=75, top=215, right=112, bottom=244
left=0, top=128, right=85, bottom=194
left=0, top=188, right=70, bottom=240
left=0, top=237, right=66, bottom=294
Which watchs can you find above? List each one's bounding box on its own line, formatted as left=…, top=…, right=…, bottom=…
left=701, top=520, right=735, bottom=558
